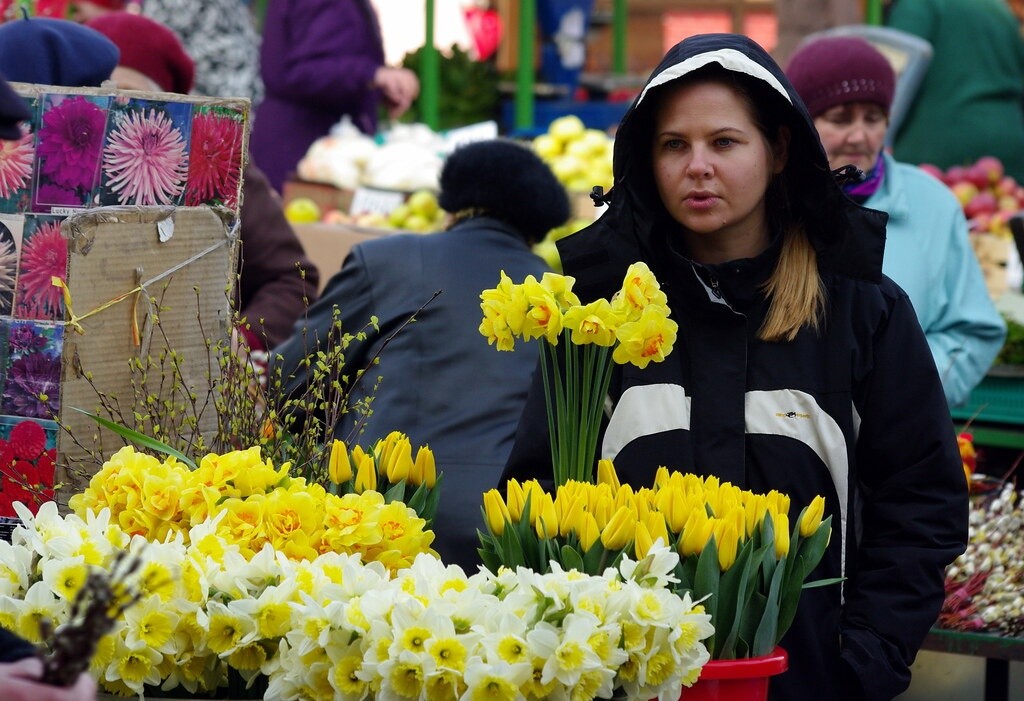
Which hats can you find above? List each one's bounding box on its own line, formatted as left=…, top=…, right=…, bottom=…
left=784, top=36, right=897, bottom=116
left=88, top=13, right=195, bottom=93
left=0, top=5, right=119, bottom=88
left=437, top=140, right=569, bottom=243
left=0, top=80, right=32, bottom=141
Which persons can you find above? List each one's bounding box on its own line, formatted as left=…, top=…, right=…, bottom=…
left=87, top=16, right=318, bottom=361
left=785, top=37, right=1006, bottom=414
left=877, top=1, right=1023, bottom=297
left=253, top=0, right=421, bottom=198
left=0, top=0, right=139, bottom=97
left=259, top=139, right=573, bottom=579
left=496, top=33, right=970, bottom=701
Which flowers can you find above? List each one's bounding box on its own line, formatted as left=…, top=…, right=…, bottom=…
left=0, top=98, right=245, bottom=518
left=1, top=273, right=717, bottom=701
left=471, top=261, right=839, bottom=658
